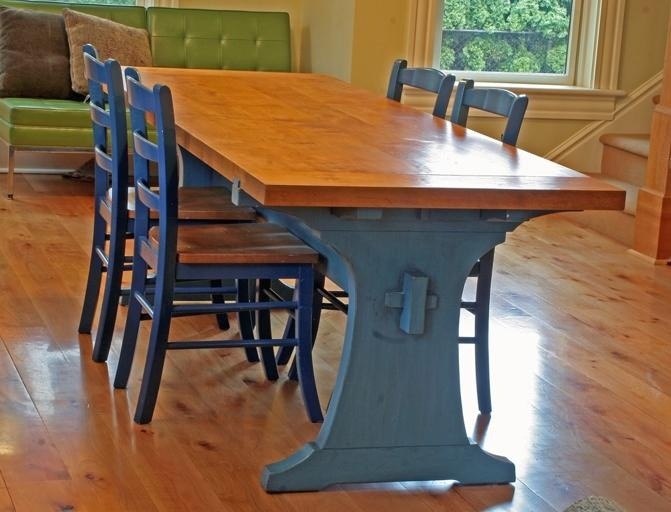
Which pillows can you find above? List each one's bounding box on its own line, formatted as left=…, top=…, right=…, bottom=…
left=61, top=7, right=152, bottom=97
left=0, top=6, right=76, bottom=98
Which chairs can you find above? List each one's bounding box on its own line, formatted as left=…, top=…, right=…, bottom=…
left=81, top=43, right=260, bottom=362
left=385, top=59, right=456, bottom=119
left=451, top=78, right=529, bottom=415
left=113, top=66, right=326, bottom=423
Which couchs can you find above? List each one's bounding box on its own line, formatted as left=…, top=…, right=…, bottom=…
left=1, top=0, right=292, bottom=198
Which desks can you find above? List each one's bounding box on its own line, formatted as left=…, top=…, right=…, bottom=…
left=100, top=65, right=626, bottom=493
